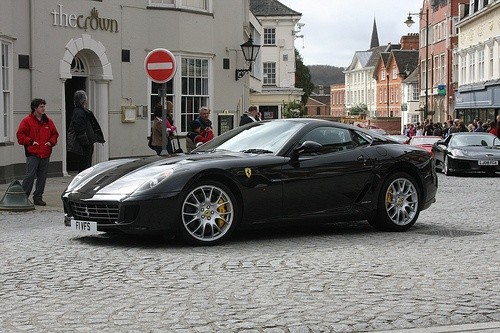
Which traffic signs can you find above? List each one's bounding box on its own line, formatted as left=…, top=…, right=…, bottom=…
left=144, top=49, right=178, bottom=84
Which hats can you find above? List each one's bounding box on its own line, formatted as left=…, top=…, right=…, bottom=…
left=190, top=120, right=202, bottom=129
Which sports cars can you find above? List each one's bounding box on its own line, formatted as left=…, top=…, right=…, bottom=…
left=431, top=132, right=500, bottom=177
left=60, top=115, right=439, bottom=244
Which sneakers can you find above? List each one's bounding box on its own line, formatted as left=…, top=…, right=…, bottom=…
left=34, top=199, right=46, bottom=206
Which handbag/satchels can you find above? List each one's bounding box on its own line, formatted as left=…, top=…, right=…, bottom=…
left=173, top=148, right=183, bottom=153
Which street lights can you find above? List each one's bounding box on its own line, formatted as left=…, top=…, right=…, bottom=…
left=404, top=9, right=432, bottom=122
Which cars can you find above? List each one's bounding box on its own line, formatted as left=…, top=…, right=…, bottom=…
left=407, top=134, right=442, bottom=160
left=370, top=128, right=410, bottom=144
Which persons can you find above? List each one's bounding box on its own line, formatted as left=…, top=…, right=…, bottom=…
left=66, top=90, right=106, bottom=173
left=159, top=101, right=183, bottom=154
left=402, top=115, right=500, bottom=139
left=186, top=119, right=214, bottom=153
left=195, top=106, right=212, bottom=139
left=16, top=98, right=60, bottom=206
left=239, top=106, right=262, bottom=126
left=148, top=104, right=171, bottom=154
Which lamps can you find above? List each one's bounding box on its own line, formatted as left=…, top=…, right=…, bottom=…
left=235, top=32, right=261, bottom=81
